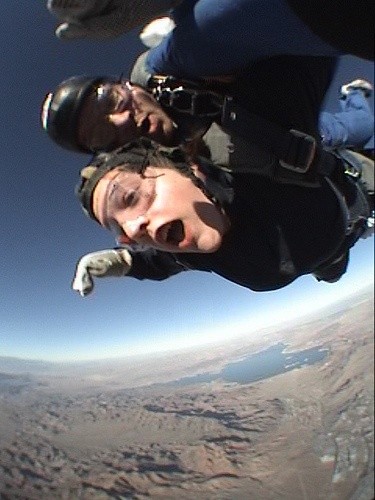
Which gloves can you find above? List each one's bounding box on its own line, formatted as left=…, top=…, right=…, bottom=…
left=48, top=1, right=186, bottom=43
left=70, top=248, right=132, bottom=297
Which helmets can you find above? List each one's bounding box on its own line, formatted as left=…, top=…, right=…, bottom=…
left=40, top=74, right=119, bottom=155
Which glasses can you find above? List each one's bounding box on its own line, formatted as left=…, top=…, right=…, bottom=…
left=105, top=172, right=154, bottom=249
left=90, top=86, right=118, bottom=154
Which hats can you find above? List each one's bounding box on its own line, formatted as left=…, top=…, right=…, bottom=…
left=71, top=137, right=183, bottom=227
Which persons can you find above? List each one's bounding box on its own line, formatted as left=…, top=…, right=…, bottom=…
left=45, top=2, right=375, bottom=297
left=40, top=1, right=375, bottom=154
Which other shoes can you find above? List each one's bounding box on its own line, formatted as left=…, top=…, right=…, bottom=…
left=340, top=78, right=373, bottom=95
